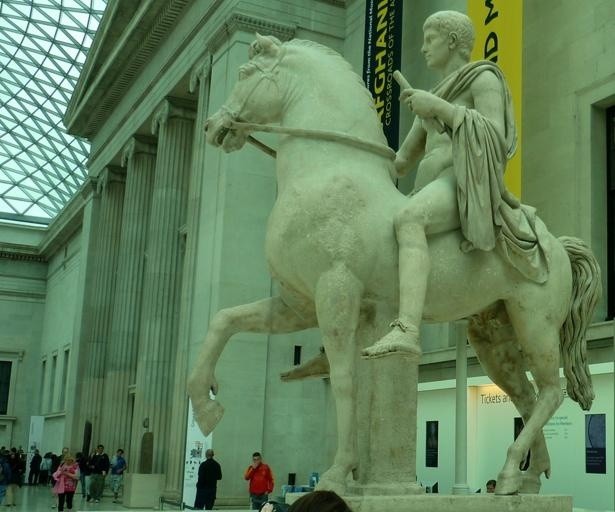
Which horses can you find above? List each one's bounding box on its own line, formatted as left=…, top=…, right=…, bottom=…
left=185, top=29, right=604, bottom=498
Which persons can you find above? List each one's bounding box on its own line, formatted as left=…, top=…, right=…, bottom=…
left=486, top=480, right=496, bottom=493
left=0, top=445, right=126, bottom=512
left=280, top=10, right=551, bottom=382
left=194, top=449, right=222, bottom=510
left=245, top=452, right=273, bottom=510
left=287, top=490, right=352, bottom=512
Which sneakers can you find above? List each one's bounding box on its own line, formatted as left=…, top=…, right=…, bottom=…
left=51, top=503, right=58, bottom=508
left=89, top=497, right=99, bottom=504
left=5, top=504, right=16, bottom=507
